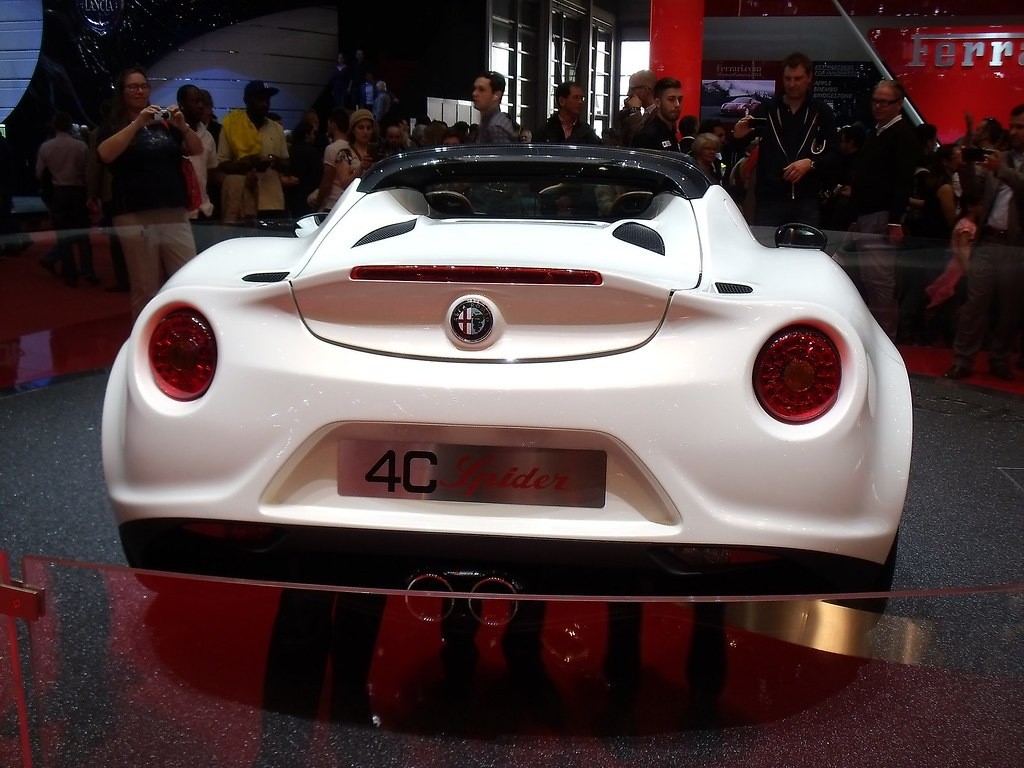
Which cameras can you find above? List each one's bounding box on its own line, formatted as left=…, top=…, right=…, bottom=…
left=153, top=110, right=172, bottom=121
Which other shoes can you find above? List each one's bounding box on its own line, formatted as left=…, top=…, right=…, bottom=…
left=945, top=364, right=972, bottom=380
left=104, top=284, right=132, bottom=292
left=989, top=361, right=1014, bottom=381
left=62, top=274, right=79, bottom=289
left=39, top=259, right=56, bottom=274
left=84, top=275, right=104, bottom=283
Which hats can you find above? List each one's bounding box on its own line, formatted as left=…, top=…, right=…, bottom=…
left=349, top=109, right=374, bottom=131
left=242, top=80, right=280, bottom=103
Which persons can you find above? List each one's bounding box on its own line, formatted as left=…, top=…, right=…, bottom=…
left=0, top=49, right=1024, bottom=379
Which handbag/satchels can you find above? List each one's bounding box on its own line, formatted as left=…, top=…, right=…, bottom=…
left=161, top=119, right=202, bottom=213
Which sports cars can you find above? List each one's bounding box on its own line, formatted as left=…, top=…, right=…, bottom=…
left=100, top=142, right=915, bottom=714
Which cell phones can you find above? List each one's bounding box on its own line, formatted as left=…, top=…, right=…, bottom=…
left=747, top=119, right=768, bottom=128
left=961, top=147, right=992, bottom=163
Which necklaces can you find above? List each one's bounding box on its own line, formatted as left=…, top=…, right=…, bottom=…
left=350, top=144, right=361, bottom=160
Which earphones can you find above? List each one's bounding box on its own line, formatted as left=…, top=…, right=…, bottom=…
left=492, top=95, right=498, bottom=101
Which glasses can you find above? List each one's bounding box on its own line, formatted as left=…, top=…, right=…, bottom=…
left=629, top=85, right=651, bottom=93
left=488, top=71, right=502, bottom=93
left=871, top=98, right=903, bottom=108
left=985, top=117, right=999, bottom=126
left=123, top=83, right=152, bottom=92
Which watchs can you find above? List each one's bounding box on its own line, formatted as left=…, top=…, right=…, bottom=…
left=810, top=158, right=815, bottom=169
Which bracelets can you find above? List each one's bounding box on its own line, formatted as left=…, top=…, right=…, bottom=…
left=629, top=107, right=641, bottom=113
left=180, top=123, right=189, bottom=131
